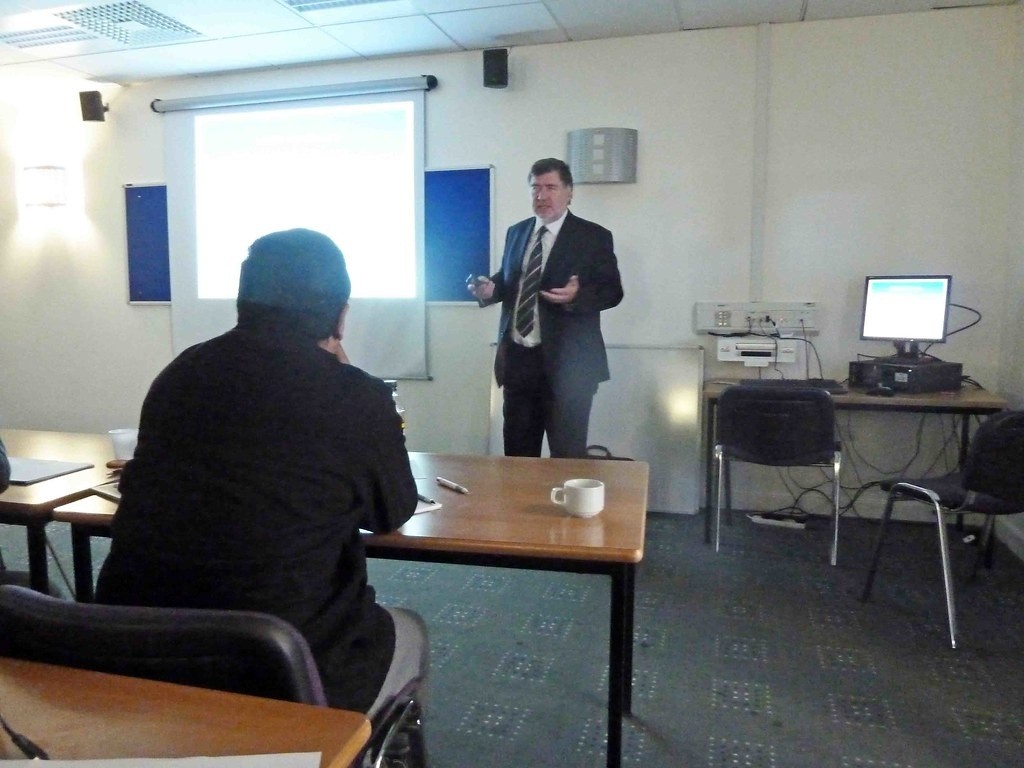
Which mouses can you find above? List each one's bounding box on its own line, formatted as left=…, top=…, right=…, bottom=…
left=865, top=387, right=894, bottom=397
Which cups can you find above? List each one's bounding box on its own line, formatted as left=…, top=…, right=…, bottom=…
left=108, top=429, right=139, bottom=460
left=551, top=479, right=605, bottom=518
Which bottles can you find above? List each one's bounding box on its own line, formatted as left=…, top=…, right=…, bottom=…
left=383, top=380, right=405, bottom=436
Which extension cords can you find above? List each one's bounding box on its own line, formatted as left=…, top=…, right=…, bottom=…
left=752, top=515, right=806, bottom=529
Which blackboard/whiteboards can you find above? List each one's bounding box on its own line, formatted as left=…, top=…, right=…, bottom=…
left=123, top=163, right=494, bottom=305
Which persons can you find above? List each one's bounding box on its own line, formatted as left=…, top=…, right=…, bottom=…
left=95, top=224, right=432, bottom=768
left=467, top=157, right=624, bottom=460
left=0, top=436, right=67, bottom=600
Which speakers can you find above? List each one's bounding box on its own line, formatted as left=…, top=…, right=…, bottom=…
left=80, top=91, right=105, bottom=122
left=483, top=49, right=509, bottom=89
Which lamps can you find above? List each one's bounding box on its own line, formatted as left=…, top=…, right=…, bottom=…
left=566, top=127, right=637, bottom=185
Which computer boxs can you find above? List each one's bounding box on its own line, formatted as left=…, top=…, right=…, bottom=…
left=847, top=360, right=962, bottom=393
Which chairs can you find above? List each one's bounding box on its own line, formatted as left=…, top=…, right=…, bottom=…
left=0, top=584, right=425, bottom=768
left=861, top=411, right=1024, bottom=649
left=714, top=385, right=843, bottom=563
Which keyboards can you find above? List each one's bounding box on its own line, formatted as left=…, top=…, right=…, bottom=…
left=740, top=378, right=847, bottom=394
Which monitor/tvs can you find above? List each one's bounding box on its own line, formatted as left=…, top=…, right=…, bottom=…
left=859, top=275, right=952, bottom=364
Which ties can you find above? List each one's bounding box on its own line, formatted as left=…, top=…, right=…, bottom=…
left=515, top=226, right=549, bottom=338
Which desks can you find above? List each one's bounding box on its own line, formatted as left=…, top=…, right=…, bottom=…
left=0, top=657, right=371, bottom=768
left=705, top=376, right=1008, bottom=566
left=0, top=429, right=121, bottom=594
left=54, top=452, right=650, bottom=768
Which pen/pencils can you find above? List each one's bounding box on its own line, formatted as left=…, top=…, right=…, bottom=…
left=437, top=477, right=469, bottom=494
left=418, top=495, right=436, bottom=504
